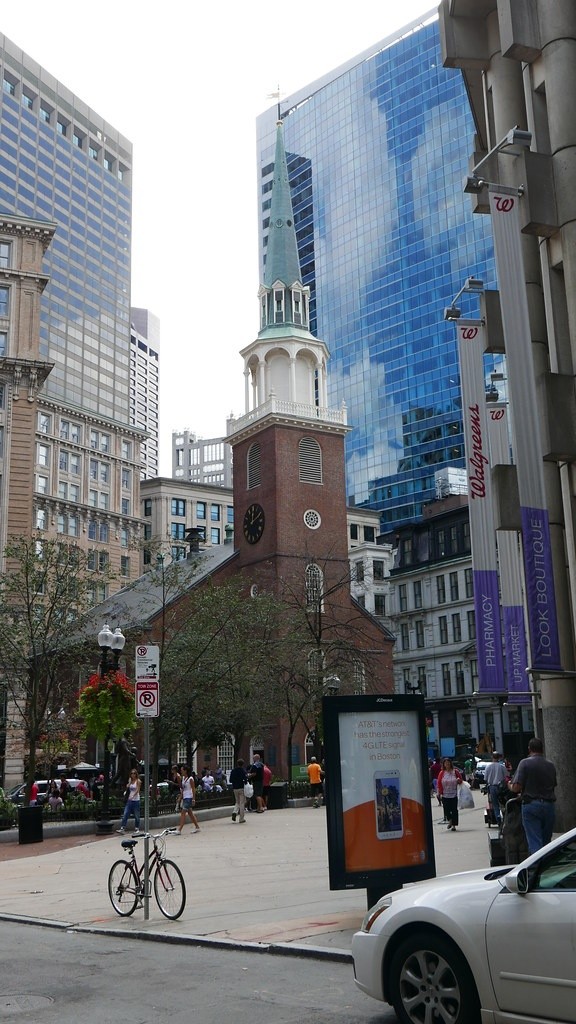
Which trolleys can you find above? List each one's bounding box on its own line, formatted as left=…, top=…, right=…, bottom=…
left=488, top=802, right=504, bottom=828
left=499, top=797, right=530, bottom=865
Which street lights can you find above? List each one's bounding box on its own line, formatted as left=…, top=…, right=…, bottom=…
left=93, top=619, right=127, bottom=834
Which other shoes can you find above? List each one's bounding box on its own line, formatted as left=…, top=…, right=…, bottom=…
left=451, top=827, right=457, bottom=831
left=312, top=802, right=320, bottom=808
left=239, top=819, right=246, bottom=823
left=231, top=811, right=237, bottom=821
left=447, top=824, right=452, bottom=829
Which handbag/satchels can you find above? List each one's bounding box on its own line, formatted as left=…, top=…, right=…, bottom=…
left=123, top=782, right=131, bottom=799
left=457, top=780, right=475, bottom=811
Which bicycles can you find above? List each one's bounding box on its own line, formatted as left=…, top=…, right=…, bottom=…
left=108, top=827, right=186, bottom=920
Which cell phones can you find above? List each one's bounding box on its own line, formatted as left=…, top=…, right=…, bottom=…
left=373, top=769, right=404, bottom=840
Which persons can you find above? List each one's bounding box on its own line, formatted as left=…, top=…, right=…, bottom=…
left=192, top=771, right=199, bottom=788
left=116, top=769, right=142, bottom=834
left=306, top=756, right=325, bottom=808
left=246, top=754, right=274, bottom=813
left=130, top=747, right=144, bottom=774
left=229, top=759, right=249, bottom=823
left=464, top=755, right=475, bottom=789
left=171, top=765, right=181, bottom=790
left=118, top=727, right=136, bottom=794
left=215, top=764, right=222, bottom=781
left=507, top=738, right=557, bottom=856
left=428, top=757, right=463, bottom=831
left=30, top=779, right=39, bottom=806
left=483, top=751, right=512, bottom=826
left=90, top=775, right=104, bottom=801
left=221, top=774, right=228, bottom=790
left=201, top=767, right=215, bottom=787
left=172, top=766, right=201, bottom=834
left=45, top=773, right=73, bottom=812
left=75, top=781, right=89, bottom=799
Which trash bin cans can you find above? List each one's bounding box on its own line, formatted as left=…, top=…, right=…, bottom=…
left=269, top=782, right=289, bottom=809
left=17, top=805, right=43, bottom=844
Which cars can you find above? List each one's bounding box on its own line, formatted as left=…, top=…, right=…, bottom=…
left=351, top=824, right=576, bottom=1024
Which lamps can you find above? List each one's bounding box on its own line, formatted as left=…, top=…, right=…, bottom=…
left=462, top=174, right=485, bottom=194
left=486, top=391, right=499, bottom=402
left=443, top=307, right=461, bottom=320
left=465, top=278, right=484, bottom=289
left=507, top=128, right=533, bottom=145
left=491, top=373, right=503, bottom=381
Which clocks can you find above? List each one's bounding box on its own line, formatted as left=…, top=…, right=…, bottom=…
left=243, top=503, right=265, bottom=545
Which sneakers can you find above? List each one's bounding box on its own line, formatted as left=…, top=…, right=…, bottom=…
left=193, top=827, right=202, bottom=833
left=171, top=830, right=181, bottom=835
left=116, top=826, right=125, bottom=835
left=133, top=827, right=140, bottom=834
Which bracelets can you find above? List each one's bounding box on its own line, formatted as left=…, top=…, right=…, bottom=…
left=192, top=800, right=195, bottom=801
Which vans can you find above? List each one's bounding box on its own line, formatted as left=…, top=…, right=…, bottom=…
left=7, top=778, right=90, bottom=805
